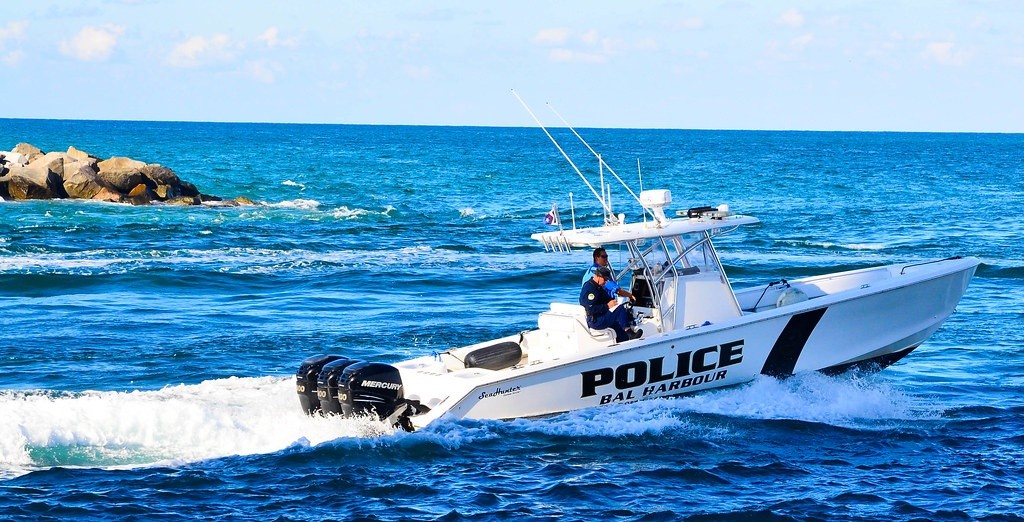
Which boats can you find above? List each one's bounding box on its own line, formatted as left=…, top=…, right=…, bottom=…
left=295, top=87, right=985, bottom=433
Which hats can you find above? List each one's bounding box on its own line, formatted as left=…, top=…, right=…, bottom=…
left=593, top=267, right=612, bottom=280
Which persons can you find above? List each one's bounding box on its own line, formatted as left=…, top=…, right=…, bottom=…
left=582, top=247, right=638, bottom=301
left=580, top=266, right=643, bottom=341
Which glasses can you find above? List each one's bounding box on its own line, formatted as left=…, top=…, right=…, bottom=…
left=599, top=255, right=608, bottom=258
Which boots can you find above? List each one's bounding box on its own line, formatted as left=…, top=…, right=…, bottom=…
left=625, top=328, right=643, bottom=340
